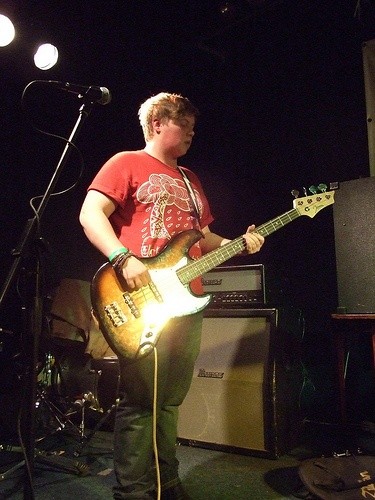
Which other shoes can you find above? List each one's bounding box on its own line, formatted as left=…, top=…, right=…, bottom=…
left=153, top=483, right=192, bottom=500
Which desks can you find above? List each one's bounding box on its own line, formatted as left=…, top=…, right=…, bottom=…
left=331, top=313, right=375, bottom=431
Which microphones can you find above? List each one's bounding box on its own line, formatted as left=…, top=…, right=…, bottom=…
left=48, top=80, right=113, bottom=106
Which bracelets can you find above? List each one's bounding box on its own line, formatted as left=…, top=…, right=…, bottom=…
left=113, top=252, right=134, bottom=271
left=109, top=246, right=128, bottom=261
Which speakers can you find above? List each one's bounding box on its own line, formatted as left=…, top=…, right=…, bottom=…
left=175, top=308, right=290, bottom=459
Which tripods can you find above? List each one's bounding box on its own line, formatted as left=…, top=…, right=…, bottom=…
left=0, top=101, right=120, bottom=483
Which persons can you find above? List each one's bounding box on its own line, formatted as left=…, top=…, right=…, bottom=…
left=80, top=92, right=264, bottom=500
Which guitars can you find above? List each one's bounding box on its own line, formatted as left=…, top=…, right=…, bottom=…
left=90, top=184, right=336, bottom=359
